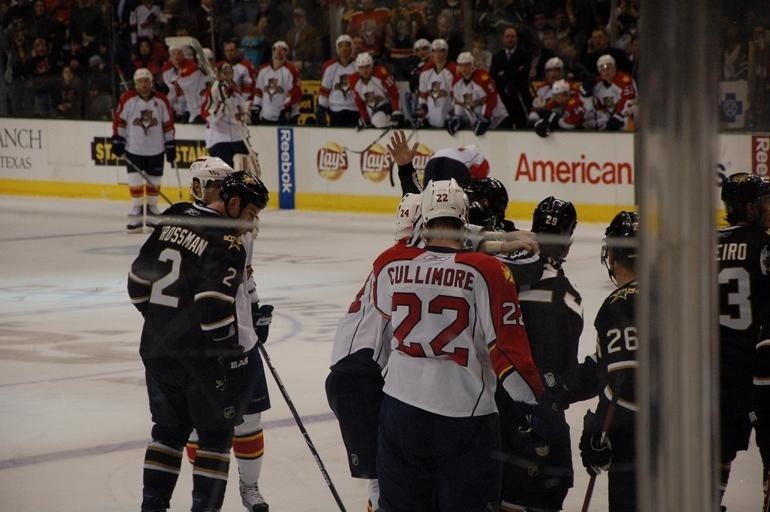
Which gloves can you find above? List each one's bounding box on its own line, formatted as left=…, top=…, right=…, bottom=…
left=216, top=344, right=246, bottom=398
left=252, top=304, right=273, bottom=343
left=579, top=428, right=611, bottom=474
left=165, top=140, right=176, bottom=162
left=543, top=354, right=608, bottom=404
left=445, top=115, right=489, bottom=135
left=111, top=135, right=126, bottom=157
left=533, top=114, right=555, bottom=137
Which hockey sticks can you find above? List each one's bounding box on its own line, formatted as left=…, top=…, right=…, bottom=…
left=390, top=130, right=416, bottom=187
left=165, top=36, right=263, bottom=181
left=342, top=128, right=391, bottom=154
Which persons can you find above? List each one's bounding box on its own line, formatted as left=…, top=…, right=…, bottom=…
left=111, top=68, right=177, bottom=235
left=127, top=157, right=274, bottom=512
left=2, top=1, right=769, bottom=131
left=331, top=129, right=768, bottom=512
left=201, top=61, right=250, bottom=169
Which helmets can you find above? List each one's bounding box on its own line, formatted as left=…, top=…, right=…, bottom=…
left=134, top=68, right=154, bottom=80
left=273, top=41, right=288, bottom=49
left=336, top=35, right=353, bottom=53
left=188, top=156, right=269, bottom=209
left=197, top=48, right=214, bottom=59
left=356, top=53, right=373, bottom=67
left=456, top=52, right=474, bottom=64
left=720, top=172, right=770, bottom=204
left=545, top=57, right=570, bottom=95
left=532, top=196, right=576, bottom=234
left=597, top=54, right=615, bottom=67
left=414, top=38, right=448, bottom=49
left=396, top=179, right=508, bottom=227
left=606, top=210, right=639, bottom=262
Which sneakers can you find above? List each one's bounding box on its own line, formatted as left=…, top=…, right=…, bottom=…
left=127, top=211, right=161, bottom=228
left=239, top=479, right=268, bottom=508
